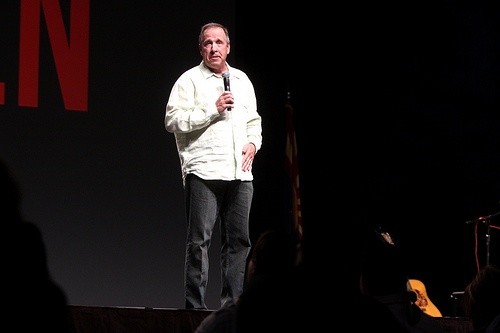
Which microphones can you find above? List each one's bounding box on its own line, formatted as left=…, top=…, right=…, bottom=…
left=222, top=71, right=232, bottom=111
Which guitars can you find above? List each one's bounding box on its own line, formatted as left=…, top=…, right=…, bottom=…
left=376, top=223, right=444, bottom=318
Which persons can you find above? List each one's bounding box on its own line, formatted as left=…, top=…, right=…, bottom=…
left=0, top=159, right=500, bottom=333
left=165, top=22, right=263, bottom=309
